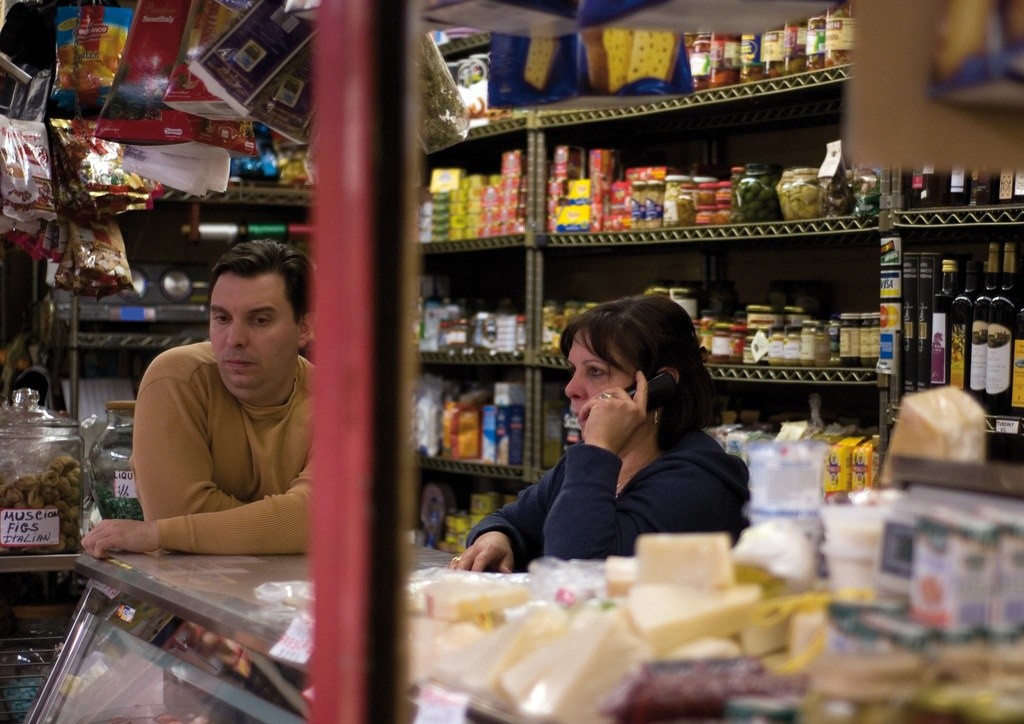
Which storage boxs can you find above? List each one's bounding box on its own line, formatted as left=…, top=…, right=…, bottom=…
left=89, top=0, right=315, bottom=158
left=421, top=147, right=529, bottom=240
left=545, top=147, right=680, bottom=235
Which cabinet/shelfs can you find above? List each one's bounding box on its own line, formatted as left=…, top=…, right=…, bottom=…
left=534, top=53, right=892, bottom=503
left=407, top=108, right=533, bottom=555
left=29, top=177, right=313, bottom=422
left=890, top=162, right=1024, bottom=471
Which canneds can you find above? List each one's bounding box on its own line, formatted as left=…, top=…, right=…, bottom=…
left=648, top=287, right=883, bottom=369
left=680, top=1, right=857, bottom=90
left=628, top=166, right=731, bottom=230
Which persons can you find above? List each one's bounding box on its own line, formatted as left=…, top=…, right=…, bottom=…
left=80, top=239, right=317, bottom=558
left=445, top=296, right=751, bottom=574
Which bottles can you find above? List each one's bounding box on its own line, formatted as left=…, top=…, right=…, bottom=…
left=0, top=387, right=86, bottom=555
left=89, top=401, right=145, bottom=522
left=911, top=158, right=1024, bottom=415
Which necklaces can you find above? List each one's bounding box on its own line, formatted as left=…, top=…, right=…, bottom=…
left=617, top=480, right=626, bottom=488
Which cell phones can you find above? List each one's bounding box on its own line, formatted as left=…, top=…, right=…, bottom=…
left=624, top=371, right=678, bottom=412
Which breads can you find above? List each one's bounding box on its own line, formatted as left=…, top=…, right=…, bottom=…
left=881, top=385, right=987, bottom=481
left=415, top=529, right=765, bottom=724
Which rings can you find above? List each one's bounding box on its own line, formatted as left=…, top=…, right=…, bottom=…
left=599, top=393, right=615, bottom=399
left=450, top=556, right=461, bottom=565
